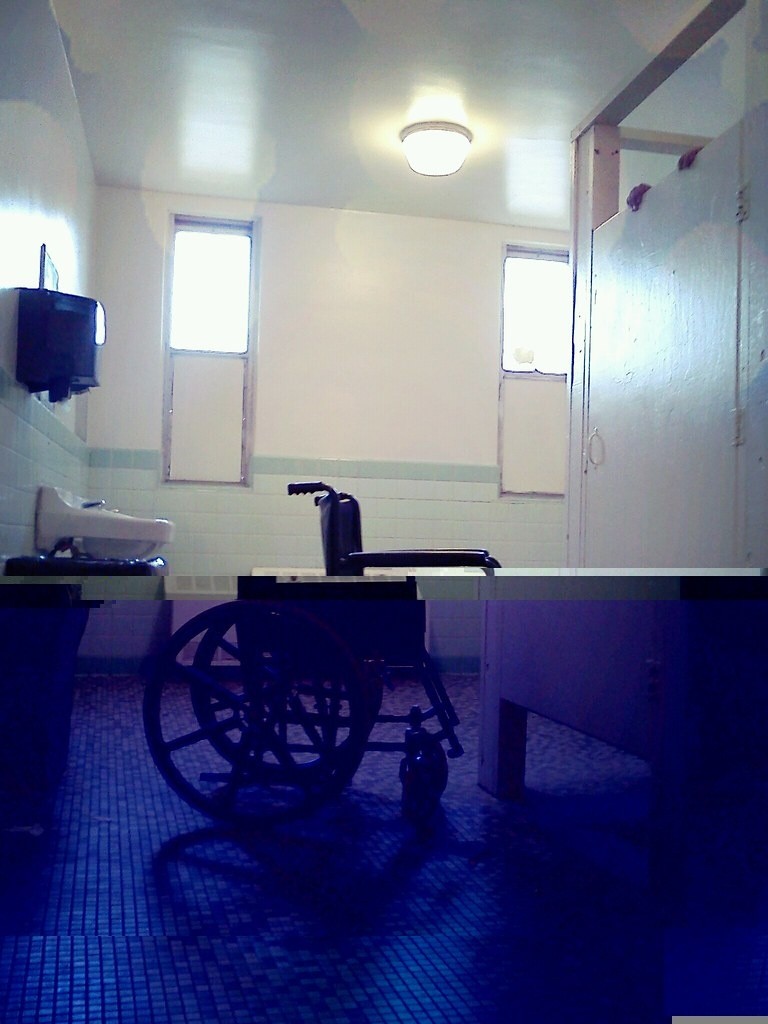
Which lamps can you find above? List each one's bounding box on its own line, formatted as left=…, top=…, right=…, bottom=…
left=399, top=122, right=473, bottom=177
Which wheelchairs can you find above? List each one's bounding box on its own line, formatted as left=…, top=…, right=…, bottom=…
left=141, top=481, right=502, bottom=829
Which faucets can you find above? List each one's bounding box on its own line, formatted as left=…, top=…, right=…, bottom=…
left=80, top=498, right=107, bottom=511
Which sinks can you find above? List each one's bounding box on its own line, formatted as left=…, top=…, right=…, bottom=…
left=34, top=484, right=176, bottom=562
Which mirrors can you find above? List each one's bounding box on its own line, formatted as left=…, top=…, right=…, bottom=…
left=37, top=244, right=60, bottom=415
